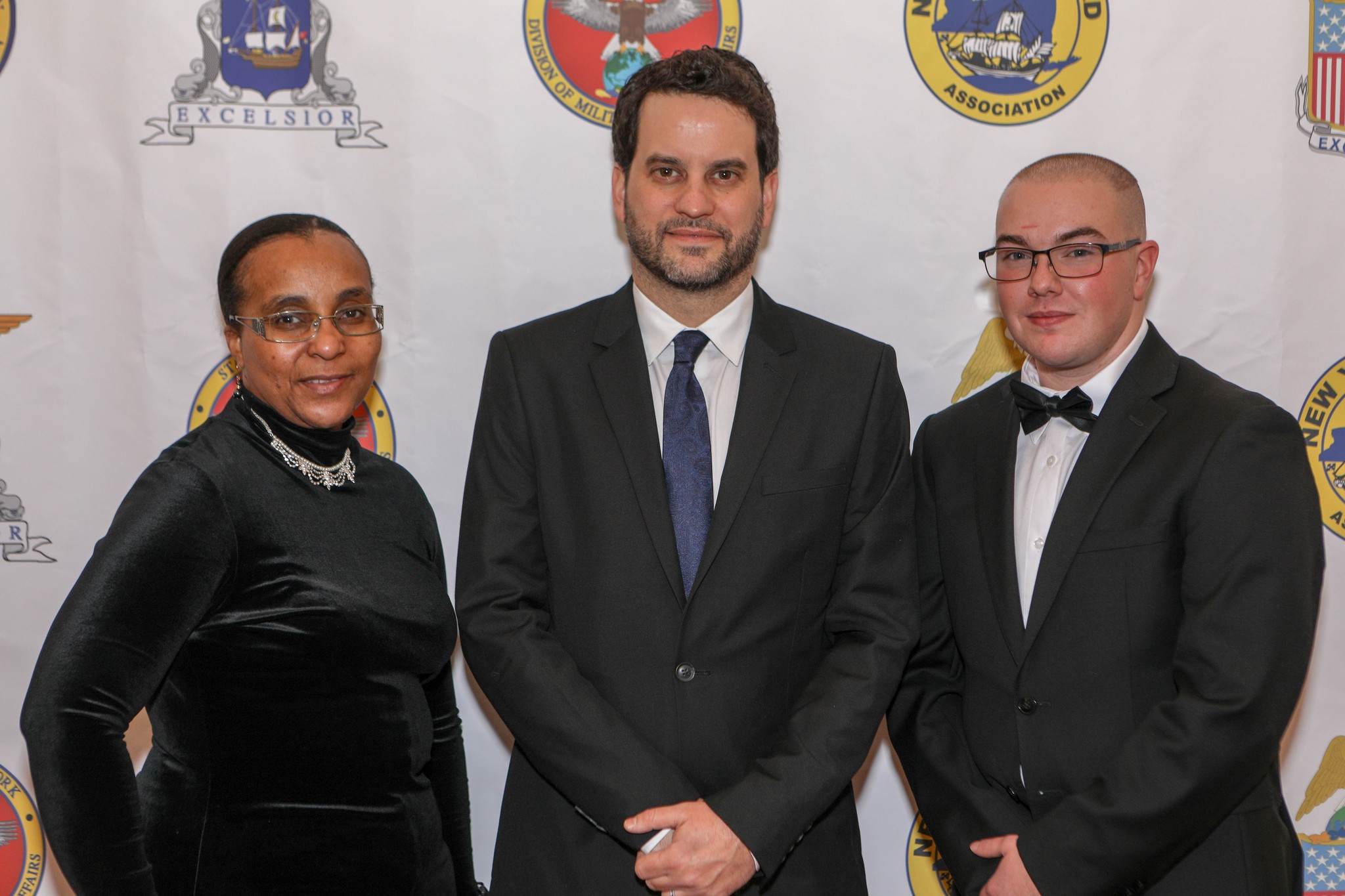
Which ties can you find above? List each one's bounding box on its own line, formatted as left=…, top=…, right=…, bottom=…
left=663, top=329, right=715, bottom=601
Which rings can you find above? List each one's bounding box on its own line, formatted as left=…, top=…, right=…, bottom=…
left=670, top=890, right=674, bottom=896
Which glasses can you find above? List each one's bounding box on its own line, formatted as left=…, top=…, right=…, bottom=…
left=229, top=304, right=383, bottom=344
left=978, top=238, right=1142, bottom=282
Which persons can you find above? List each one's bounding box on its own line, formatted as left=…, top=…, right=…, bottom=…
left=886, top=153, right=1325, bottom=896
left=19, top=212, right=484, bottom=896
left=454, top=45, right=924, bottom=896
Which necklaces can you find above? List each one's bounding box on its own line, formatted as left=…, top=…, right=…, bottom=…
left=242, top=396, right=356, bottom=490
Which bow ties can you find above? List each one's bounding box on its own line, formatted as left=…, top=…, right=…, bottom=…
left=1010, top=379, right=1098, bottom=436
